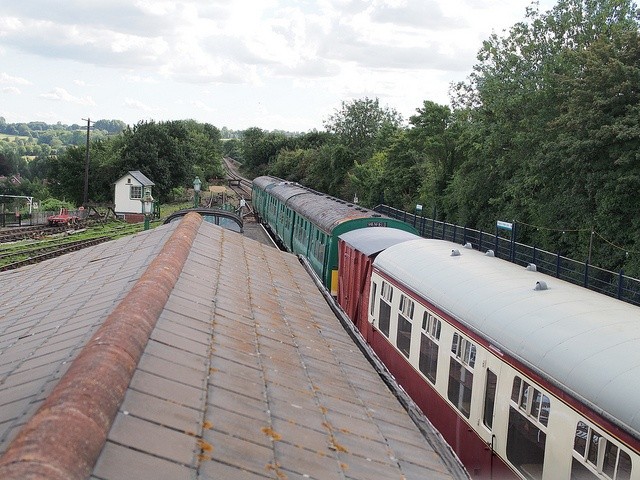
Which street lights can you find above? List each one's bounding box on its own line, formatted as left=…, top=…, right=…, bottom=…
left=140, top=192, right=154, bottom=230
left=193, top=176, right=202, bottom=208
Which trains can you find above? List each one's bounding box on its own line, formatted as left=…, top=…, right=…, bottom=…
left=251, top=175, right=640, bottom=479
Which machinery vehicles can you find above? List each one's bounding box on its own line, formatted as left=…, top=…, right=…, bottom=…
left=47, top=206, right=79, bottom=227
